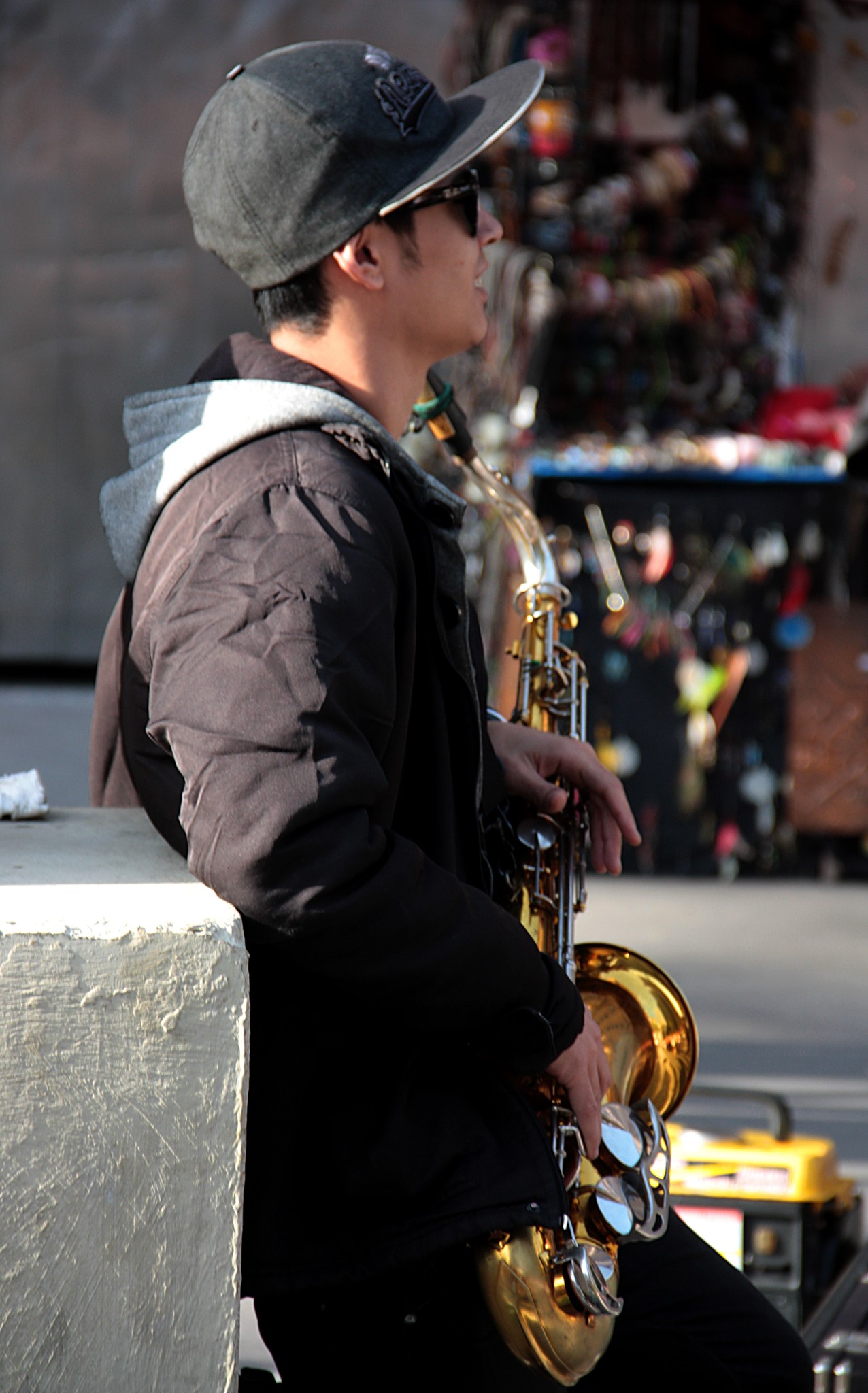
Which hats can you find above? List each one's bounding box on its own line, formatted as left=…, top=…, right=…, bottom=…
left=181, top=38, right=544, bottom=287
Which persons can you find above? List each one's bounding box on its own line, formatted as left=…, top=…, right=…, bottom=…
left=86, top=40, right=817, bottom=1393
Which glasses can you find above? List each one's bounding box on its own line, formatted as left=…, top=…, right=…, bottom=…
left=394, top=168, right=481, bottom=240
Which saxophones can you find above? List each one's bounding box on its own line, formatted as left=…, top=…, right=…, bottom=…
left=400, top=367, right=698, bottom=1392
